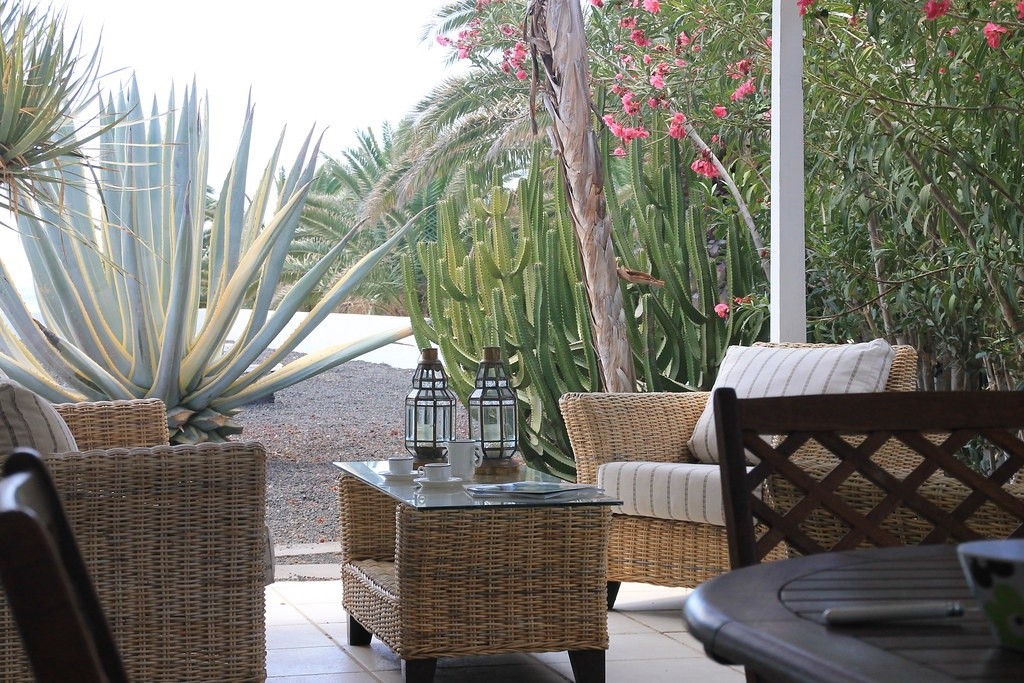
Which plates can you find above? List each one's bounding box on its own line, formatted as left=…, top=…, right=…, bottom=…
left=375, top=470, right=423, bottom=480
left=412, top=477, right=462, bottom=487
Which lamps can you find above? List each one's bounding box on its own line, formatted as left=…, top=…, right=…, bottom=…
left=467, top=346, right=521, bottom=475
left=404, top=348, right=457, bottom=469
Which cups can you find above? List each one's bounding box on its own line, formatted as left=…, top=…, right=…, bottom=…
left=956, top=539, right=1024, bottom=650
left=417, top=463, right=451, bottom=480
left=387, top=457, right=413, bottom=472
left=443, top=439, right=484, bottom=482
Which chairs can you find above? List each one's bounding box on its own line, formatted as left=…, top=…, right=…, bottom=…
left=0, top=398, right=274, bottom=683
left=0, top=446, right=130, bottom=683
left=558, top=341, right=1024, bottom=612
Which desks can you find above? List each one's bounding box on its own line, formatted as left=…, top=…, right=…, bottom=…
left=681, top=544, right=1024, bottom=683
left=334, top=461, right=625, bottom=683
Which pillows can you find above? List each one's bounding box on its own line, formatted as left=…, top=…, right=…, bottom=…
left=688, top=338, right=894, bottom=465
left=0, top=367, right=80, bottom=453
